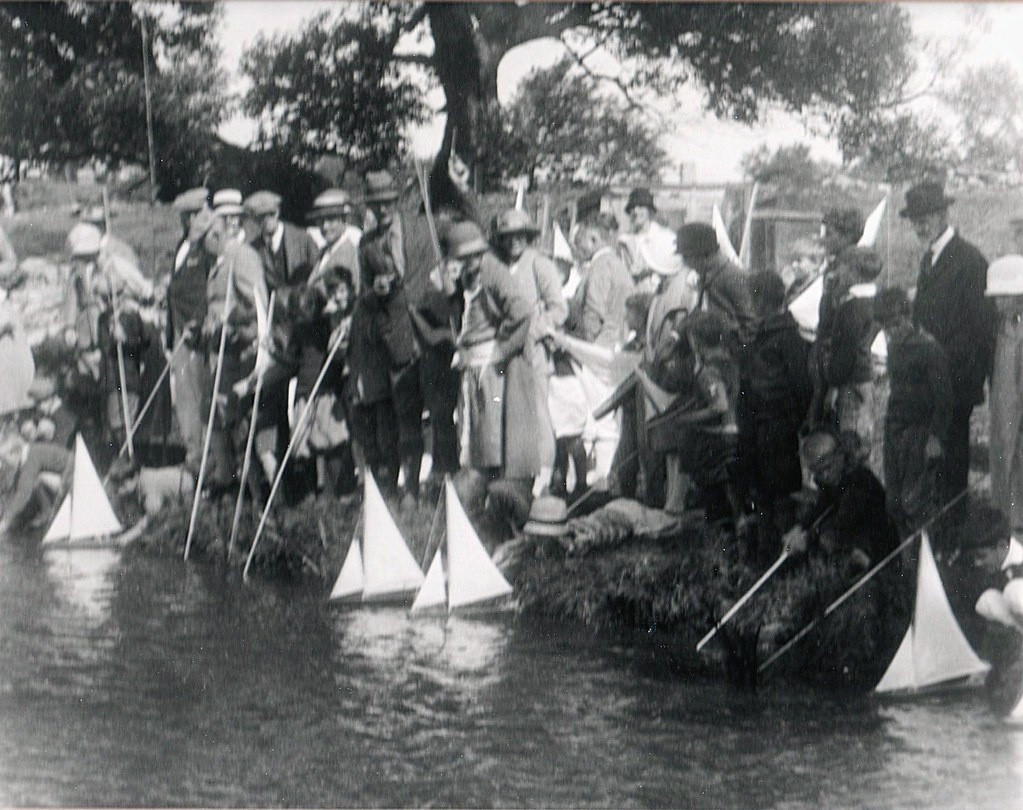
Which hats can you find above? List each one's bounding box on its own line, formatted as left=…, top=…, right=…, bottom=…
left=303, top=189, right=350, bottom=220
left=685, top=309, right=723, bottom=346
left=445, top=218, right=490, bottom=258
left=170, top=186, right=208, bottom=213
left=823, top=205, right=864, bottom=237
left=625, top=187, right=657, bottom=215
left=242, top=190, right=282, bottom=216
left=488, top=209, right=541, bottom=244
left=212, top=188, right=244, bottom=219
left=63, top=221, right=101, bottom=262
left=673, top=223, right=720, bottom=254
left=362, top=170, right=399, bottom=204
left=522, top=493, right=569, bottom=537
left=899, top=185, right=957, bottom=219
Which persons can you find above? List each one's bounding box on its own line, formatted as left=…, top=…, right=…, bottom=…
left=0, top=380, right=77, bottom=536
left=872, top=285, right=955, bottom=534
left=110, top=457, right=195, bottom=548
left=62, top=204, right=155, bottom=448
left=960, top=506, right=1023, bottom=631
left=451, top=467, right=534, bottom=542
left=900, top=184, right=1000, bottom=518
left=783, top=427, right=902, bottom=575
left=156, top=179, right=882, bottom=565
